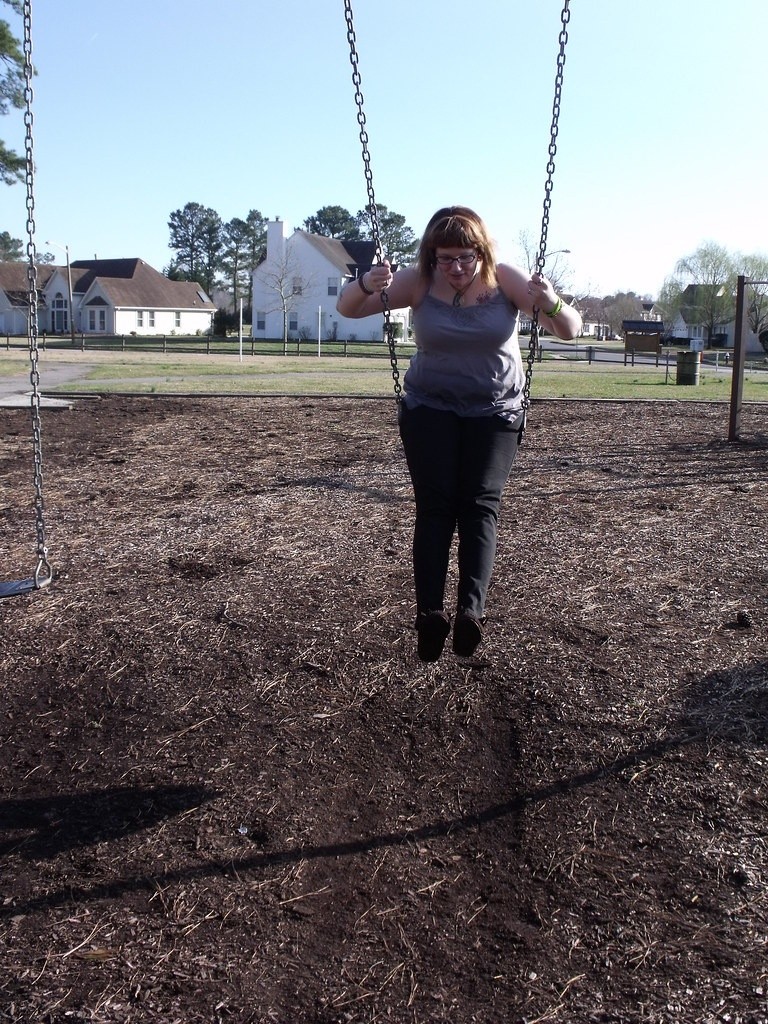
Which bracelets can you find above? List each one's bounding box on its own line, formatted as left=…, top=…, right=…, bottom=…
left=358, top=272, right=374, bottom=295
left=543, top=297, right=563, bottom=318
left=363, top=272, right=370, bottom=291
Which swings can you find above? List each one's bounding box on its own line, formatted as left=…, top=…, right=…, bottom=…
left=343, top=0, right=580, bottom=457
left=0, top=0, right=61, bottom=600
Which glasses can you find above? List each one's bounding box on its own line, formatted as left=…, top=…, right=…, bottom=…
left=434, top=249, right=477, bottom=263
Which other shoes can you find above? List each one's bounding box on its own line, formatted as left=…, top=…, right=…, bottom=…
left=420, top=611, right=449, bottom=663
left=453, top=613, right=483, bottom=657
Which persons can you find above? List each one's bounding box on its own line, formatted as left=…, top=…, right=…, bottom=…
left=336, top=206, right=582, bottom=663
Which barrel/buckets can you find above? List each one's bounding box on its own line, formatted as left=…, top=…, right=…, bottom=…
left=676, top=352, right=701, bottom=385
